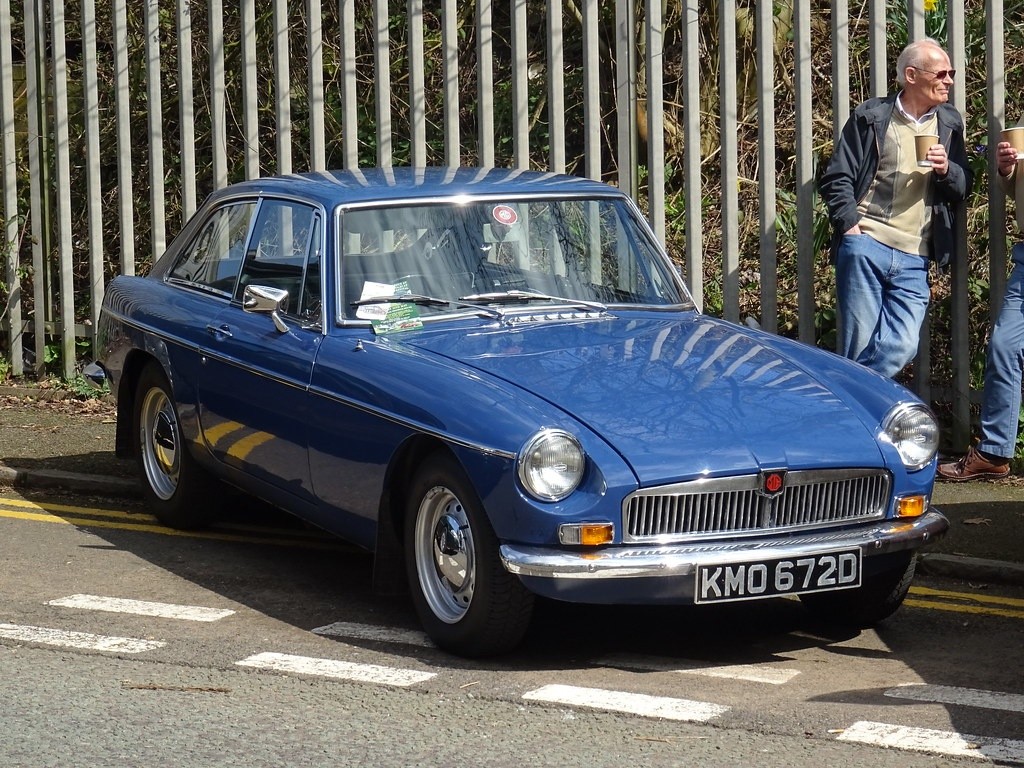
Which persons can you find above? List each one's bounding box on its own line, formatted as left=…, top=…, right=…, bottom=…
left=936, top=112, right=1024, bottom=482
left=817, top=38, right=976, bottom=378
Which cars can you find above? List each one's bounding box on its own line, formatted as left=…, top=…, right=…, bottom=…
left=81, top=167, right=949, bottom=660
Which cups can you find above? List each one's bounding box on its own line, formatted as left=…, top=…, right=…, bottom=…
left=999, top=127, right=1024, bottom=159
left=914, top=135, right=939, bottom=166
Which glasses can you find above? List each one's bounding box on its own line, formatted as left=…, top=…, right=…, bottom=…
left=903, top=66, right=956, bottom=79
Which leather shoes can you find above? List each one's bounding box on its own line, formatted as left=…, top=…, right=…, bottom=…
left=936, top=445, right=1011, bottom=482
